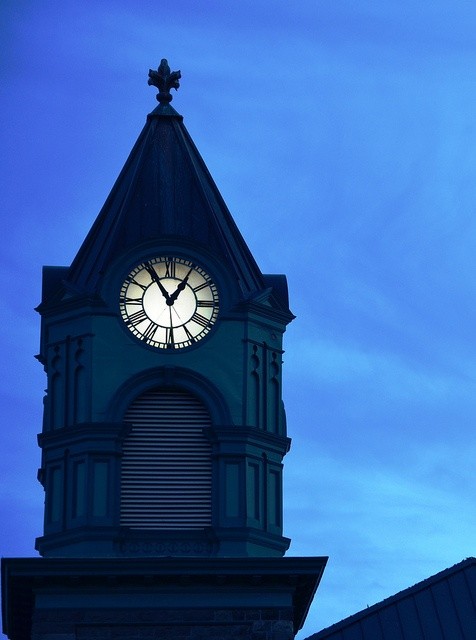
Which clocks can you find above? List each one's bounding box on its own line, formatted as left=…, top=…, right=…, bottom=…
left=119, top=254, right=220, bottom=350
